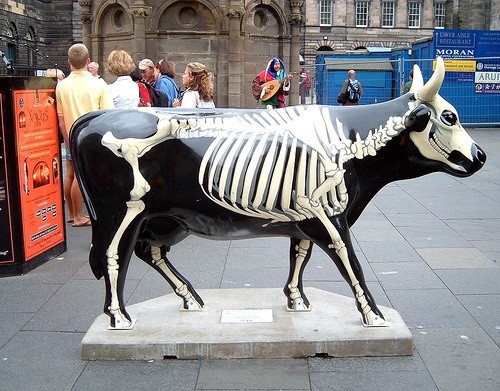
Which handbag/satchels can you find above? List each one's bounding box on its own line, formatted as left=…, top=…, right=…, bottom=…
left=337, top=91, right=346, bottom=105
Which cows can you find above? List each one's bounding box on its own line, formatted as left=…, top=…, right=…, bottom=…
left=69, top=56, right=488, bottom=331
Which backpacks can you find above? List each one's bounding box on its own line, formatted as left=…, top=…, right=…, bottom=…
left=141, top=80, right=168, bottom=107
left=347, top=79, right=360, bottom=102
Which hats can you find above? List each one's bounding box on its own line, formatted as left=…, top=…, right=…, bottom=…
left=139, top=59, right=154, bottom=70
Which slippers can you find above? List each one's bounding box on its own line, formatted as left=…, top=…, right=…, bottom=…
left=67, top=216, right=91, bottom=223
left=72, top=219, right=91, bottom=227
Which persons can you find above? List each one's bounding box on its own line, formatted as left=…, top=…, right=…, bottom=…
left=402, top=70, right=414, bottom=95
left=252, top=57, right=294, bottom=109
left=172, top=61, right=216, bottom=108
left=106, top=49, right=140, bottom=108
left=303, top=70, right=311, bottom=97
left=153, top=58, right=179, bottom=107
left=56, top=44, right=116, bottom=226
left=138, top=58, right=156, bottom=85
left=341, top=70, right=363, bottom=105
left=46, top=69, right=65, bottom=82
left=88, top=61, right=107, bottom=85
left=130, top=62, right=153, bottom=107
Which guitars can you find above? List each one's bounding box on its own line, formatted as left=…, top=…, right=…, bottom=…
left=260, top=66, right=302, bottom=102
